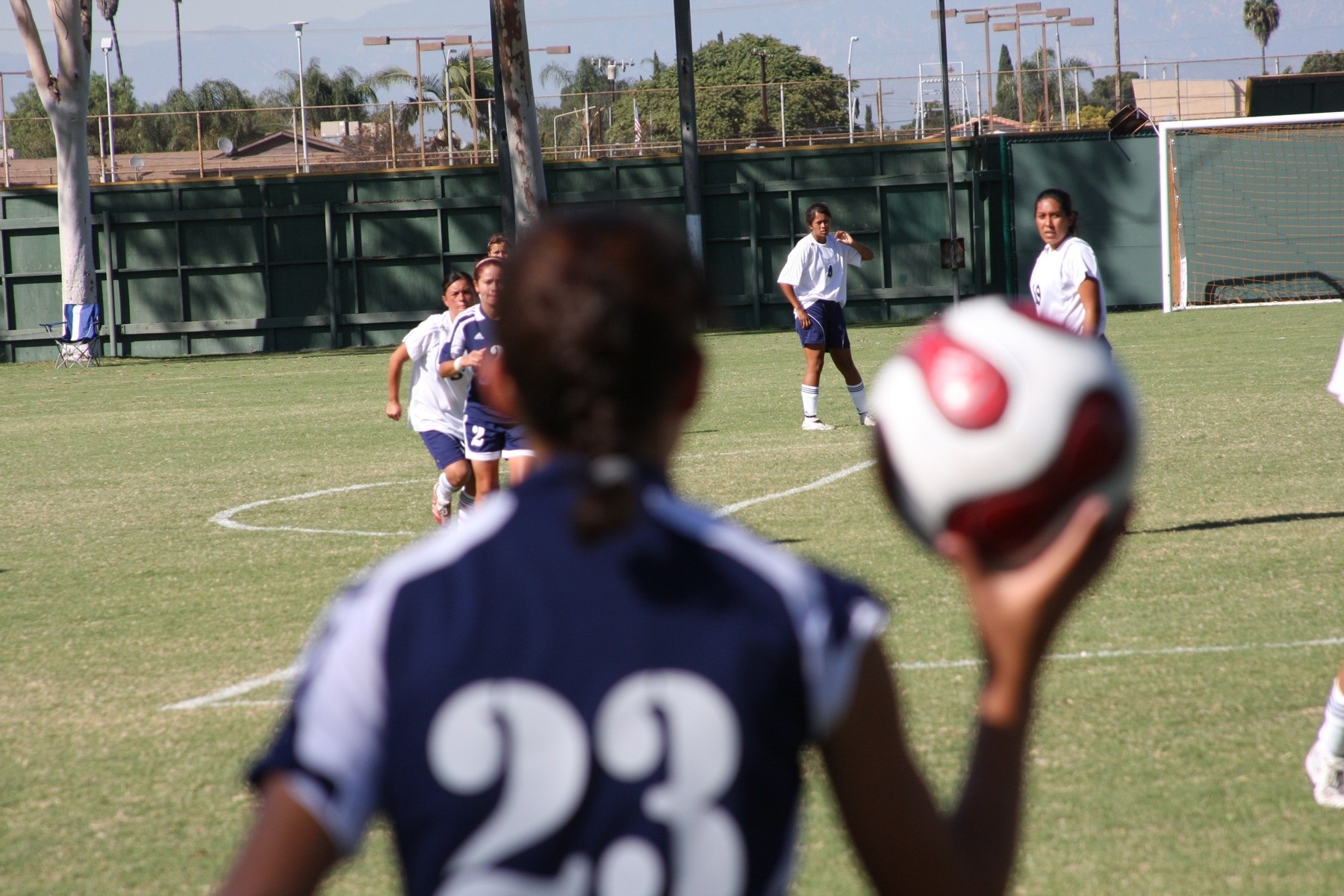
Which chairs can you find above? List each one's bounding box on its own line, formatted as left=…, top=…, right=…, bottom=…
left=39, top=303, right=103, bottom=371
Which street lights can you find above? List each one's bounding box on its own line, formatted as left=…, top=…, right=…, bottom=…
left=362, top=35, right=472, bottom=167
left=288, top=20, right=310, bottom=173
left=847, top=36, right=860, bottom=144
left=100, top=36, right=116, bottom=183
left=419, top=42, right=458, bottom=167
left=931, top=1, right=1096, bottom=132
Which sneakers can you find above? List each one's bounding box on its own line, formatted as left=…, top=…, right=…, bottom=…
left=801, top=418, right=835, bottom=431
left=1305, top=741, right=1344, bottom=808
left=860, top=414, right=877, bottom=426
left=432, top=483, right=452, bottom=525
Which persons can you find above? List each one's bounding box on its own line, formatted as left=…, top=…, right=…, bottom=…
left=1029, top=189, right=1113, bottom=359
left=777, top=204, right=878, bottom=430
left=387, top=234, right=539, bottom=526
left=208, top=203, right=1140, bottom=896
left=1305, top=337, right=1344, bottom=812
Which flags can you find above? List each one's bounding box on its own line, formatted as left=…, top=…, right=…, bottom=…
left=633, top=99, right=643, bottom=146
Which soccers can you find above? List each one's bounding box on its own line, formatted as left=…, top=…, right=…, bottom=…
left=865, top=291, right=1139, bottom=571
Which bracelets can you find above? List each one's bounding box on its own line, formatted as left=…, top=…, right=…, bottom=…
left=454, top=357, right=463, bottom=372
